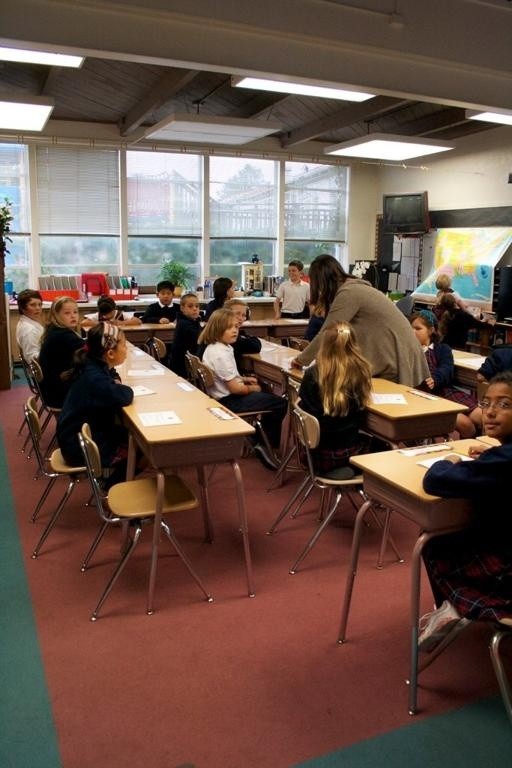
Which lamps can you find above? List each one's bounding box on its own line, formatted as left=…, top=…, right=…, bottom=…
left=0, top=46, right=87, bottom=70
left=0, top=94, right=56, bottom=135
left=144, top=113, right=282, bottom=145
left=324, top=132, right=456, bottom=161
left=231, top=74, right=379, bottom=103
left=465, top=109, right=512, bottom=126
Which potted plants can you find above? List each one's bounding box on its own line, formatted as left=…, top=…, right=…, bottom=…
left=154, top=259, right=197, bottom=295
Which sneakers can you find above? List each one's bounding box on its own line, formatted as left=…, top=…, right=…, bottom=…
left=254, top=444, right=279, bottom=470
left=414, top=600, right=459, bottom=652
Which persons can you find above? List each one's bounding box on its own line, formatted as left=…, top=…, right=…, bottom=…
left=272, top=259, right=311, bottom=347
left=290, top=254, right=431, bottom=389
left=204, top=277, right=234, bottom=322
left=143, top=280, right=181, bottom=324
left=222, top=297, right=262, bottom=378
left=470, top=346, right=512, bottom=429
left=297, top=320, right=388, bottom=480
left=55, top=322, right=147, bottom=495
left=37, top=296, right=86, bottom=408
left=409, top=309, right=476, bottom=439
left=171, top=294, right=203, bottom=376
left=438, top=294, right=496, bottom=351
left=416, top=370, right=512, bottom=653
left=304, top=289, right=326, bottom=342
left=15, top=288, right=46, bottom=364
left=434, top=273, right=468, bottom=312
left=80, top=294, right=144, bottom=326
left=196, top=309, right=288, bottom=472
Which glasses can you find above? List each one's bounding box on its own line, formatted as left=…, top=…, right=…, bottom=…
left=477, top=397, right=510, bottom=410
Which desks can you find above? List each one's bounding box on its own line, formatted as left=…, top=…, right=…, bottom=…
left=9, top=296, right=278, bottom=365
left=452, top=349, right=490, bottom=388
left=239, top=318, right=273, bottom=337
left=81, top=324, right=155, bottom=355
left=115, top=360, right=178, bottom=380
left=248, top=347, right=316, bottom=392
left=124, top=346, right=155, bottom=362
left=367, top=384, right=469, bottom=450
left=476, top=435, right=502, bottom=446
left=120, top=398, right=256, bottom=615
left=143, top=322, right=175, bottom=344
left=239, top=338, right=282, bottom=371
left=266, top=318, right=311, bottom=338
left=120, top=377, right=210, bottom=400
left=284, top=360, right=397, bottom=401
left=337, top=438, right=492, bottom=715
left=125, top=340, right=135, bottom=347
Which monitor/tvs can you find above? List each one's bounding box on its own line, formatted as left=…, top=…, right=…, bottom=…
left=382, top=191, right=431, bottom=236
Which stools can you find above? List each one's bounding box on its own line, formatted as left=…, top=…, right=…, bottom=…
left=405, top=616, right=512, bottom=718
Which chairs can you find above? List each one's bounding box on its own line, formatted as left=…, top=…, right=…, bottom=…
left=22, top=396, right=88, bottom=560
left=287, top=336, right=310, bottom=351
left=184, top=350, right=274, bottom=482
left=78, top=423, right=213, bottom=621
left=267, top=383, right=315, bottom=519
left=266, top=397, right=403, bottom=574
left=141, top=336, right=166, bottom=361
left=22, top=357, right=61, bottom=458
left=17, top=342, right=39, bottom=437
